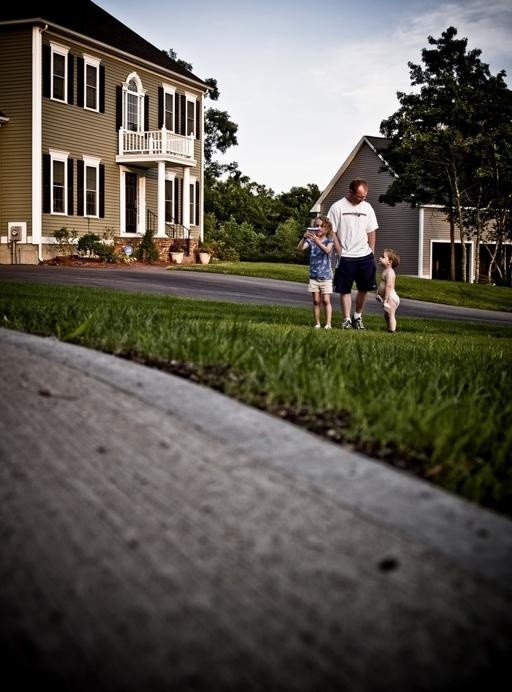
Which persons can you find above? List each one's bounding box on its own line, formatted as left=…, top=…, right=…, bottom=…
left=376, top=248, right=400, bottom=333
left=296, top=215, right=334, bottom=329
left=329, top=178, right=380, bottom=331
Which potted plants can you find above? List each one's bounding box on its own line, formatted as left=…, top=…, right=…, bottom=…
left=168, top=239, right=217, bottom=266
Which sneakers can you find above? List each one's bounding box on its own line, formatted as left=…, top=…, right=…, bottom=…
left=352, top=315, right=365, bottom=329
left=340, top=319, right=352, bottom=329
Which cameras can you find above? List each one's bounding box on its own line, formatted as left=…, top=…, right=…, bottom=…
left=306, top=227, right=322, bottom=237
